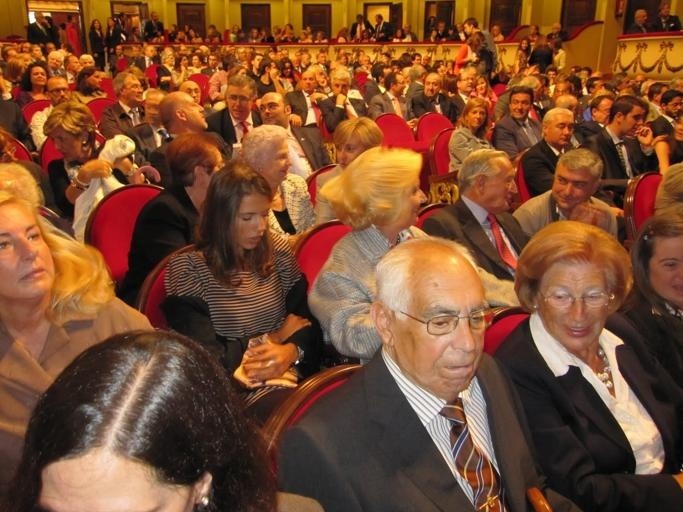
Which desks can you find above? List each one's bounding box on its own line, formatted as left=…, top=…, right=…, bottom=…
left=117, top=40, right=530, bottom=72
left=613, top=31, right=682, bottom=81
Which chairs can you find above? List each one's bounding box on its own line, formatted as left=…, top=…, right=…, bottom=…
left=471, top=309, right=529, bottom=356
left=249, top=362, right=371, bottom=512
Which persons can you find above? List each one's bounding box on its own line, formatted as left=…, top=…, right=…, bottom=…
left=0, top=3, right=682, bottom=511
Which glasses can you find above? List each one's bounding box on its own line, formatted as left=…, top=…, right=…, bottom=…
left=538, top=287, right=615, bottom=308
left=48, top=87, right=71, bottom=96
left=400, top=309, right=495, bottom=336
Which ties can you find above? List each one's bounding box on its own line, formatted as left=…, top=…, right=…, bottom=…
left=439, top=398, right=507, bottom=512
left=157, top=129, right=168, bottom=147
left=616, top=140, right=627, bottom=174
left=487, top=213, right=518, bottom=270
left=239, top=120, right=250, bottom=143
left=523, top=120, right=537, bottom=146
left=128, top=108, right=140, bottom=127
left=392, top=97, right=402, bottom=118
left=308, top=94, right=322, bottom=128
left=343, top=100, right=354, bottom=120
left=147, top=58, right=152, bottom=67
